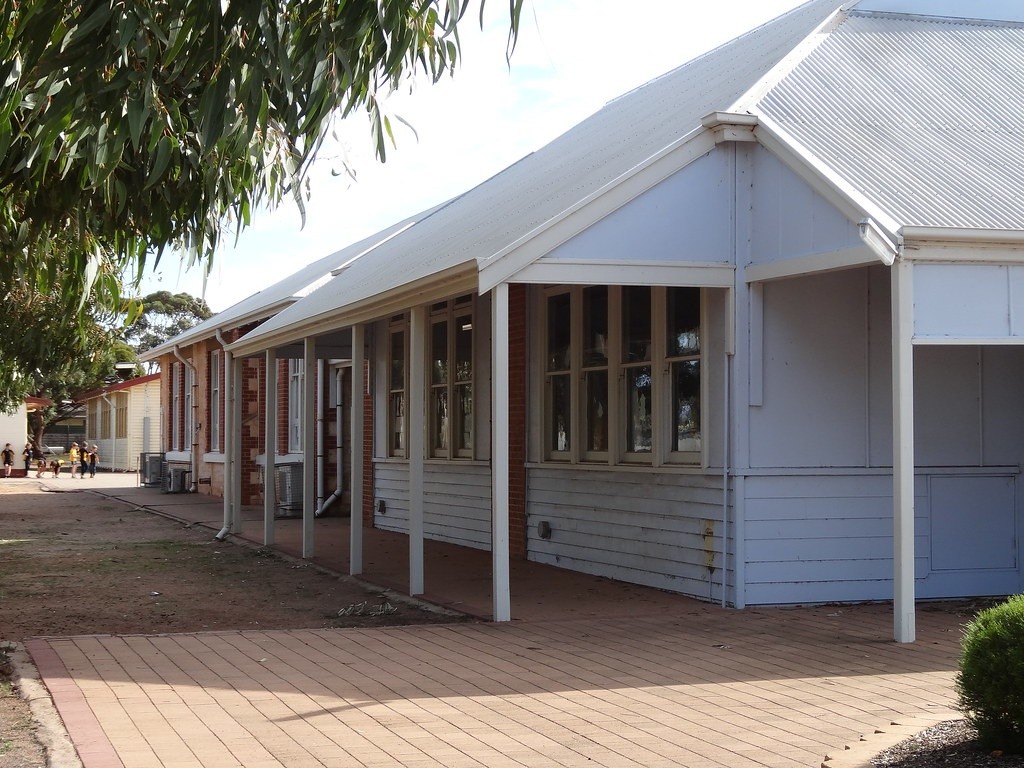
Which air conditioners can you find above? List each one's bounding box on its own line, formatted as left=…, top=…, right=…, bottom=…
left=278, top=466, right=304, bottom=510
left=168, top=469, right=186, bottom=491
left=145, top=457, right=160, bottom=482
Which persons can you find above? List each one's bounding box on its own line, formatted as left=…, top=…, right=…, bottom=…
left=35, top=458, right=47, bottom=477
left=70, top=441, right=100, bottom=478
left=22, top=443, right=34, bottom=475
left=50, top=459, right=66, bottom=477
left=1, top=443, right=15, bottom=477
